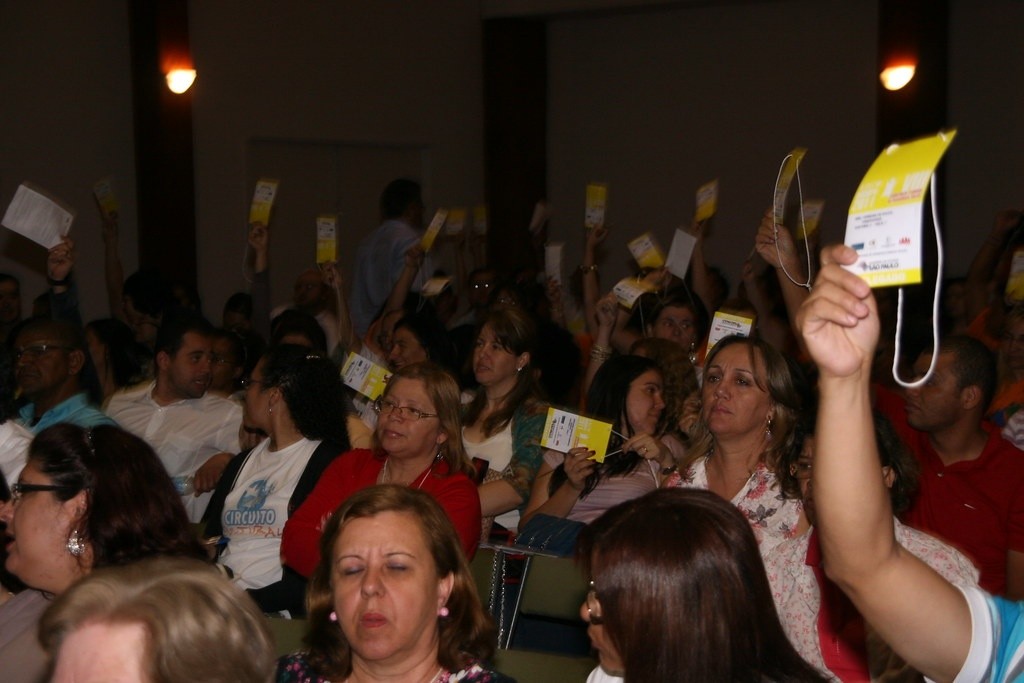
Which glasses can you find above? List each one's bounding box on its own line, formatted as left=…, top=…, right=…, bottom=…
left=9, top=483, right=68, bottom=498
left=495, top=299, right=518, bottom=307
left=469, top=280, right=492, bottom=291
left=291, top=283, right=318, bottom=290
left=211, top=356, right=235, bottom=368
left=13, top=345, right=70, bottom=360
left=585, top=579, right=606, bottom=625
left=242, top=378, right=262, bottom=389
left=374, top=399, right=440, bottom=421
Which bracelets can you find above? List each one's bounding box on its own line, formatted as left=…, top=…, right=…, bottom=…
left=590, top=344, right=612, bottom=363
left=579, top=264, right=599, bottom=274
left=661, top=464, right=677, bottom=474
left=48, top=275, right=70, bottom=286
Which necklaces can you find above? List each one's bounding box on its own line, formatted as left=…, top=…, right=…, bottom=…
left=383, top=458, right=431, bottom=490
left=429, top=667, right=442, bottom=683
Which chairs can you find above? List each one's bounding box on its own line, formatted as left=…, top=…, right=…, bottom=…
left=271, top=538, right=594, bottom=682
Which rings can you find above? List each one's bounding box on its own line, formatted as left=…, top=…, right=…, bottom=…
left=644, top=447, right=647, bottom=452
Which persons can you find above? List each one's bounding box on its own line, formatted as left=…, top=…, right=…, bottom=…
left=758, top=213, right=1024, bottom=601
left=0, top=180, right=1023, bottom=546
left=578, top=489, right=828, bottom=681
left=760, top=398, right=979, bottom=683
left=281, top=362, right=482, bottom=586
left=1, top=422, right=209, bottom=682
left=275, top=484, right=519, bottom=683
left=201, top=343, right=350, bottom=619
left=668, top=335, right=839, bottom=683
left=518, top=355, right=681, bottom=526
left=39, top=556, right=274, bottom=683
left=796, top=244, right=1024, bottom=681
left=105, top=318, right=243, bottom=523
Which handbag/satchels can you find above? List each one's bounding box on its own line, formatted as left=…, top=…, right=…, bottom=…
left=509, top=512, right=588, bottom=556
